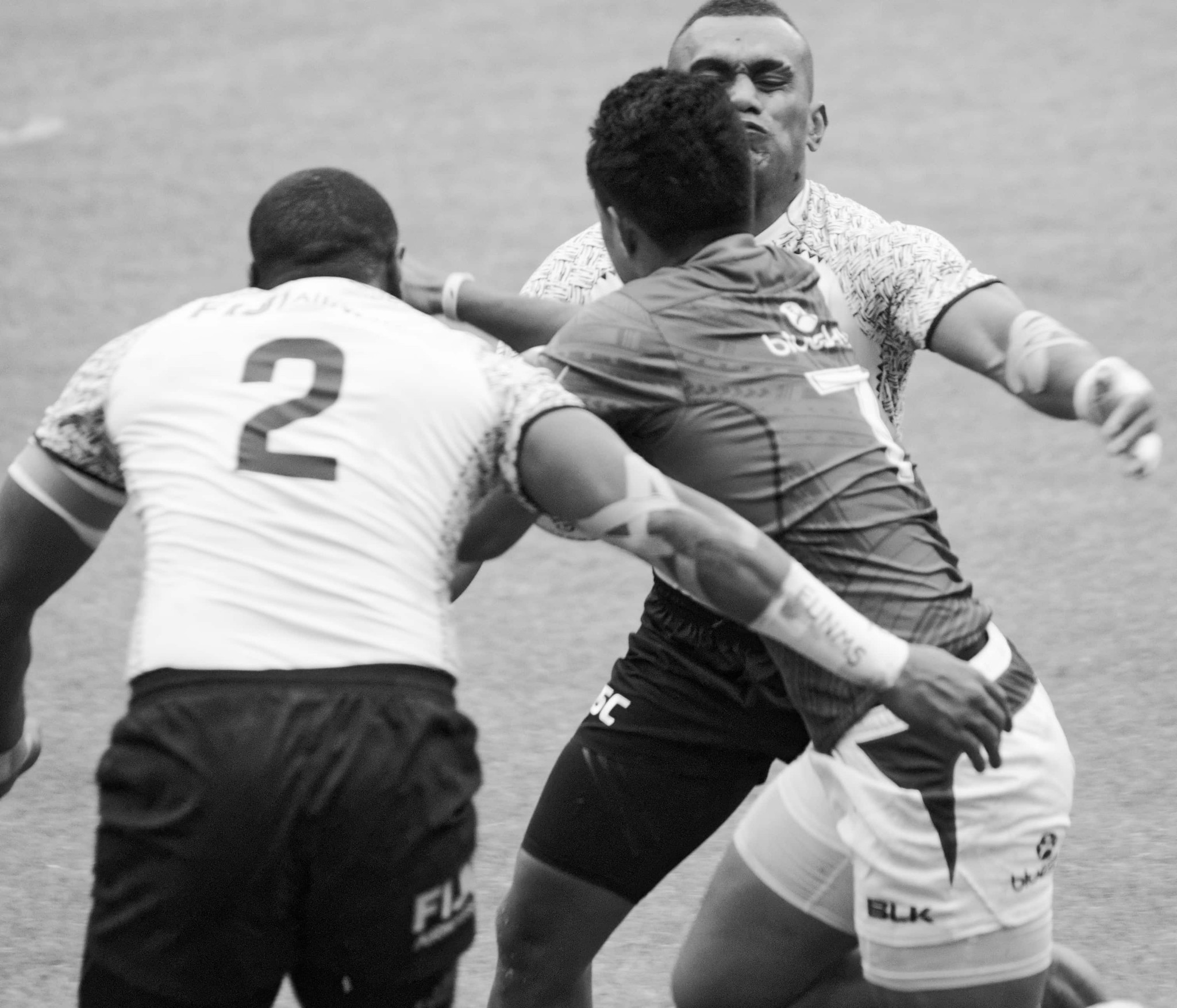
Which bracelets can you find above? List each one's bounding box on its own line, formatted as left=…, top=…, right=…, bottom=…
left=0, top=727, right=31, bottom=783
left=442, top=272, right=475, bottom=321
left=1072, top=356, right=1130, bottom=423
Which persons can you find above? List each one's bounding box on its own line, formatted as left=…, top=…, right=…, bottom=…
left=0, top=169, right=1013, bottom=1008
left=442, top=0, right=1163, bottom=1008
left=400, top=68, right=1075, bottom=1008
